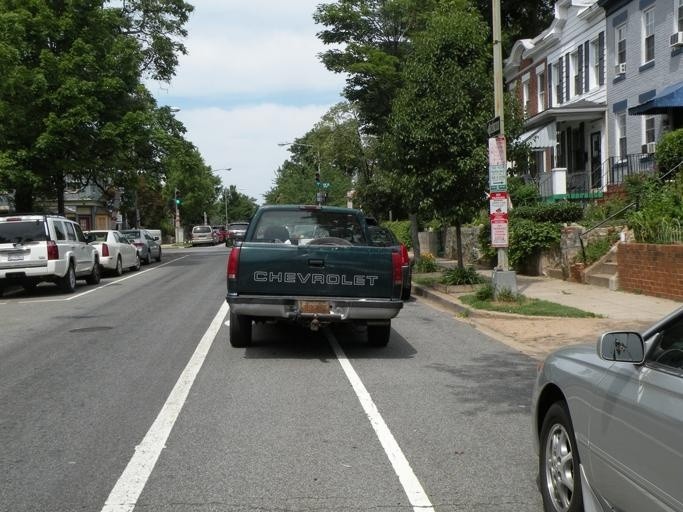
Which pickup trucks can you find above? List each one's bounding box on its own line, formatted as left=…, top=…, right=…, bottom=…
left=226, top=204, right=401, bottom=348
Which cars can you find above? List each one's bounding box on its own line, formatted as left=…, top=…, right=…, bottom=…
left=364, top=226, right=411, bottom=300
left=191, top=225, right=227, bottom=246
left=0, top=215, right=164, bottom=292
left=525, top=303, right=683, bottom=511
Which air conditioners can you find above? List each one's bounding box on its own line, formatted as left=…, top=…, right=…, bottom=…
left=669, top=32, right=683, bottom=47
left=647, top=142, right=658, bottom=153
left=614, top=63, right=626, bottom=75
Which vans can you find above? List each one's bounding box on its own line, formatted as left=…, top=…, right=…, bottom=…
left=226, top=222, right=251, bottom=247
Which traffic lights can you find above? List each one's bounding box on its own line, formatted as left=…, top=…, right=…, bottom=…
left=315, top=172, right=320, bottom=182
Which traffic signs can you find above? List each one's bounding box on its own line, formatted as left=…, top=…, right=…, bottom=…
left=317, top=182, right=330, bottom=188
left=485, top=117, right=501, bottom=137
left=176, top=190, right=181, bottom=205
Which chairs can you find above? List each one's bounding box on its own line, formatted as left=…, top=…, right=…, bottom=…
left=263, top=224, right=289, bottom=240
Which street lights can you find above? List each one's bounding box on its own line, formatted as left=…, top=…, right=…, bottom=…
left=205, top=168, right=232, bottom=175
left=278, top=142, right=323, bottom=207
